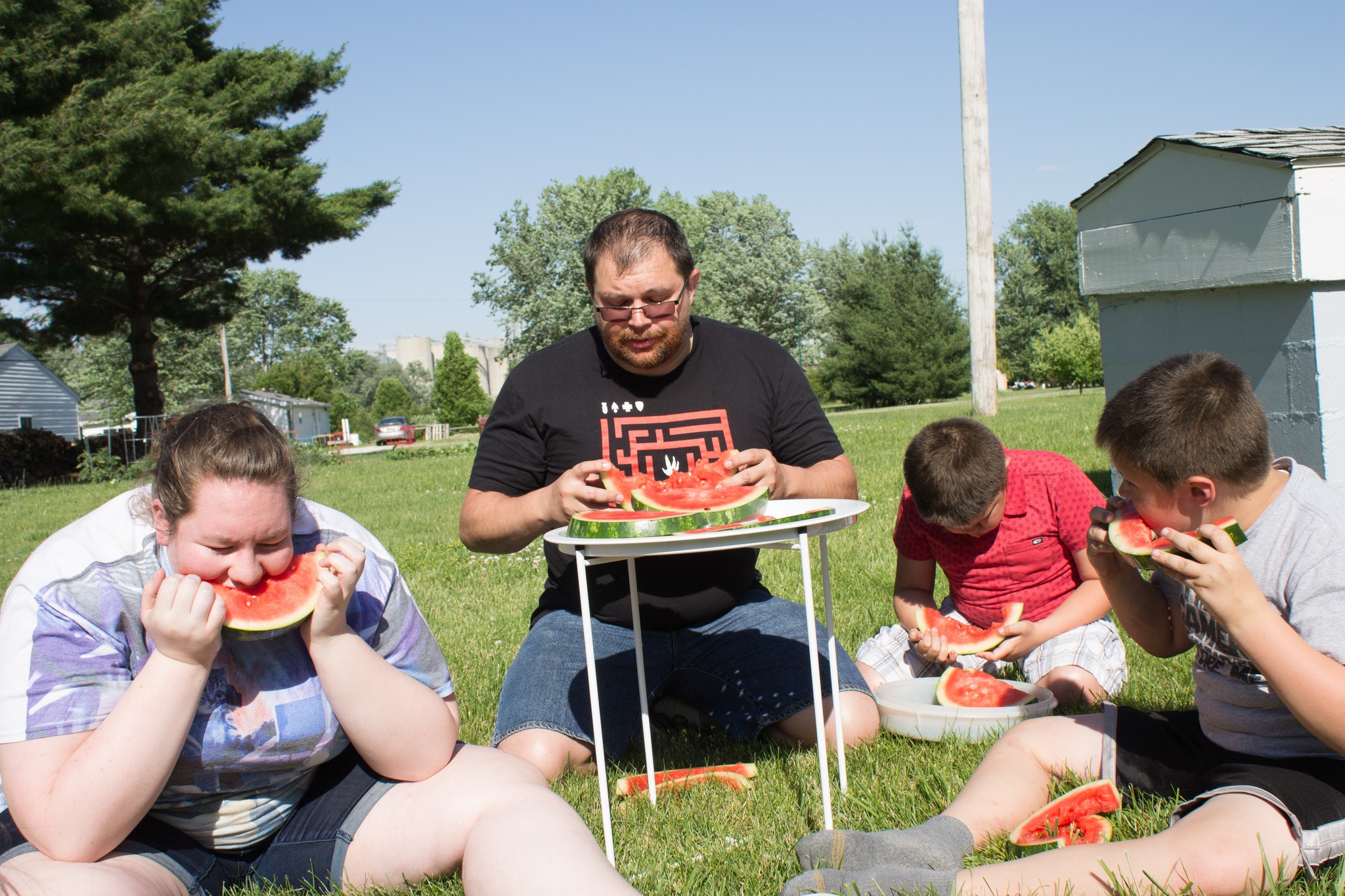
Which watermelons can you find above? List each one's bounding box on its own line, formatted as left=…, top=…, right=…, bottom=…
left=566, top=451, right=836, bottom=539
left=1109, top=498, right=1249, bottom=569
left=914, top=602, right=1025, bottom=656
left=936, top=666, right=1038, bottom=707
left=201, top=551, right=329, bottom=641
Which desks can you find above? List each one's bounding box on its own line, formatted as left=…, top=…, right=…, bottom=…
left=542, top=500, right=872, bottom=877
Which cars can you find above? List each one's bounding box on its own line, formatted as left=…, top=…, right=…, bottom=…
left=374, top=416, right=416, bottom=446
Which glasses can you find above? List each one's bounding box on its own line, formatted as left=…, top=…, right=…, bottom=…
left=591, top=279, right=688, bottom=322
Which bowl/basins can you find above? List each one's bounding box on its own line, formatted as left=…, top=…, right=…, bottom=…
left=872, top=677, right=1059, bottom=745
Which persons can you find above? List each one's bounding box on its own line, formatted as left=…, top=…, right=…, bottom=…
left=1, top=403, right=651, bottom=896
left=846, top=419, right=1128, bottom=707
left=779, top=348, right=1344, bottom=896
left=460, top=207, right=882, bottom=789
